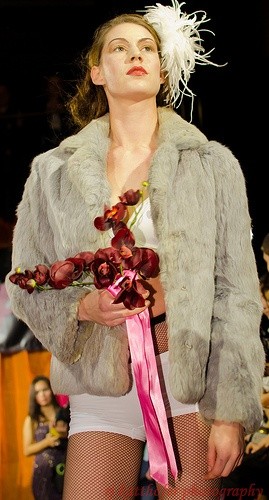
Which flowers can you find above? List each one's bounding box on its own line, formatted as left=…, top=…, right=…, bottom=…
left=9, top=190, right=161, bottom=310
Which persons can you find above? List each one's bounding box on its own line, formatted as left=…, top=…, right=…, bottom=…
left=242, top=237, right=269, bottom=458
left=5, top=12, right=266, bottom=500
left=22, top=373, right=72, bottom=500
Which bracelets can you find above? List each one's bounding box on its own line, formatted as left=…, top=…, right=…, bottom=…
left=258, top=428, right=269, bottom=434
